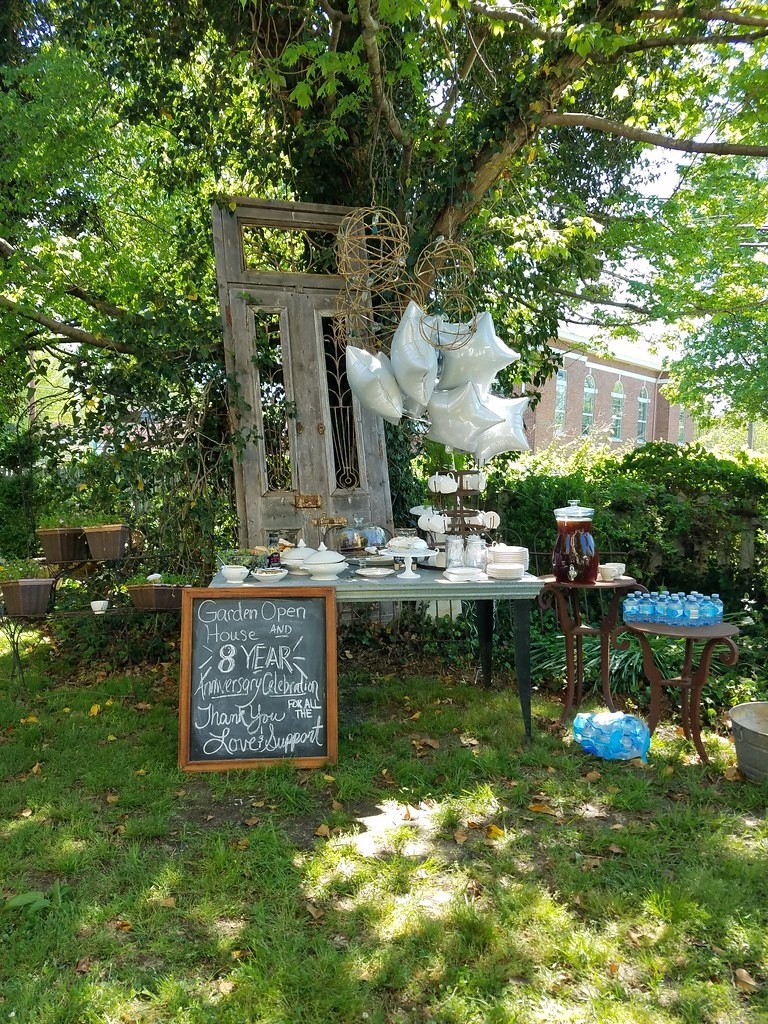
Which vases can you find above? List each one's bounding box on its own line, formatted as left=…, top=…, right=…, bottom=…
left=125, top=583, right=192, bottom=608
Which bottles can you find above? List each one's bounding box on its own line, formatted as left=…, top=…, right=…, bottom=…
left=268, top=534, right=281, bottom=568
left=552, top=500, right=598, bottom=584
left=623, top=591, right=723, bottom=626
left=622, top=730, right=632, bottom=760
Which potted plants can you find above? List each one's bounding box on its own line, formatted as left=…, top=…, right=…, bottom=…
left=82, top=510, right=129, bottom=558
left=0, top=556, right=56, bottom=615
left=35, top=506, right=84, bottom=560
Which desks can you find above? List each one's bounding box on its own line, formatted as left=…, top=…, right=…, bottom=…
left=207, top=564, right=545, bottom=737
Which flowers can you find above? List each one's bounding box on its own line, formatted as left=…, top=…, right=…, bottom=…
left=128, top=573, right=200, bottom=586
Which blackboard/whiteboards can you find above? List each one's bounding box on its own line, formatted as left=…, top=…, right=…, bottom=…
left=181, top=587, right=339, bottom=772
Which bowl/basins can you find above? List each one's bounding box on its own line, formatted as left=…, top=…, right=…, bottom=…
left=281, top=539, right=318, bottom=575
left=222, top=566, right=249, bottom=583
left=299, top=542, right=348, bottom=581
left=251, top=569, right=288, bottom=582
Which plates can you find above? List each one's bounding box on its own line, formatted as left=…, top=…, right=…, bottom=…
left=447, top=567, right=482, bottom=574
left=410, top=505, right=432, bottom=515
left=487, top=546, right=529, bottom=580
left=355, top=567, right=395, bottom=577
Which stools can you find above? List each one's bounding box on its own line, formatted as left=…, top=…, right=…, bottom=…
left=537, top=573, right=650, bottom=724
left=611, top=622, right=740, bottom=769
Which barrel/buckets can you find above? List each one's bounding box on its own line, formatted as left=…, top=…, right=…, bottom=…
left=729, top=701, right=768, bottom=786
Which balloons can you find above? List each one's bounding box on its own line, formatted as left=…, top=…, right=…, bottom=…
left=434, top=311, right=520, bottom=392
left=476, top=392, right=532, bottom=462
left=424, top=379, right=506, bottom=453
left=345, top=346, right=404, bottom=426
left=390, top=300, right=443, bottom=406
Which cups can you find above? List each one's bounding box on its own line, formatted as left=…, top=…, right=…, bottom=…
left=428, top=548, right=444, bottom=567
left=458, top=471, right=486, bottom=492
left=599, top=563, right=625, bottom=582
left=428, top=514, right=448, bottom=534
left=479, top=512, right=500, bottom=529
left=418, top=511, right=436, bottom=531
left=91, top=601, right=108, bottom=615
left=440, top=473, right=457, bottom=493
left=428, top=472, right=441, bottom=493
